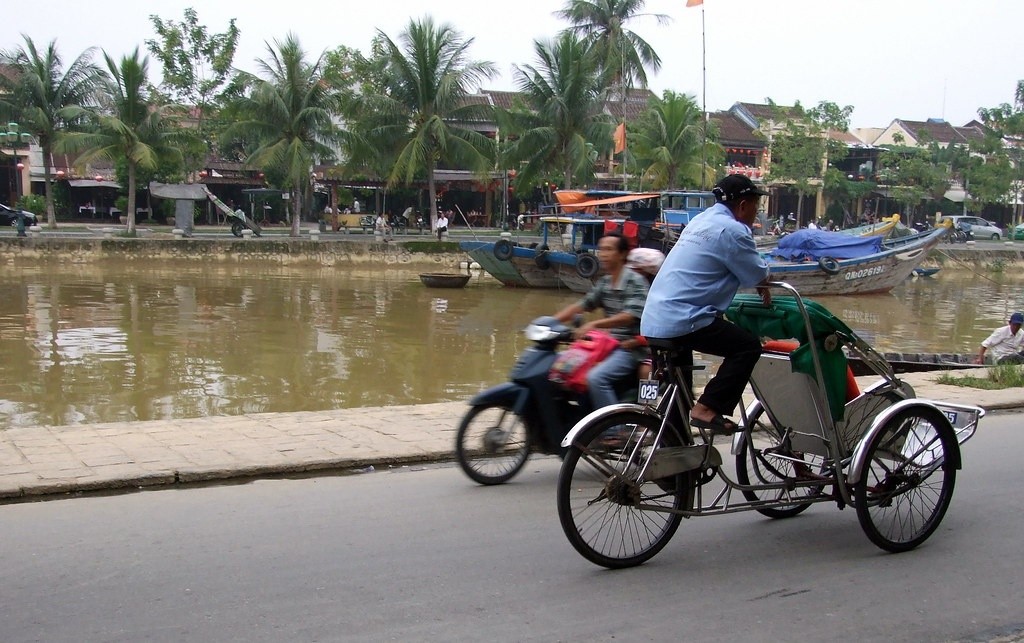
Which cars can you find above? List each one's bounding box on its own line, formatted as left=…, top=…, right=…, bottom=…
left=1007, top=222, right=1024, bottom=241
left=941, top=214, right=1004, bottom=244
left=0, top=203, right=39, bottom=227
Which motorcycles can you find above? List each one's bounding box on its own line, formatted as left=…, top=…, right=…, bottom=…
left=453, top=315, right=673, bottom=488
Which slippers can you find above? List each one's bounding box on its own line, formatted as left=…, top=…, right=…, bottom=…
left=690, top=414, right=739, bottom=432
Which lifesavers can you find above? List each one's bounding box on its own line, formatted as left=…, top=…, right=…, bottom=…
left=819, top=255, right=841, bottom=275
left=575, top=253, right=599, bottom=278
left=494, top=240, right=514, bottom=261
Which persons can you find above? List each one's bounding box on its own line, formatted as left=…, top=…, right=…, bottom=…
left=415, top=208, right=484, bottom=227
left=956, top=220, right=971, bottom=238
left=435, top=212, right=448, bottom=242
left=353, top=197, right=360, bottom=214
left=803, top=217, right=836, bottom=235
left=640, top=174, right=772, bottom=491
left=376, top=211, right=393, bottom=242
left=549, top=233, right=661, bottom=447
left=401, top=205, right=415, bottom=228
left=517, top=213, right=524, bottom=227
left=324, top=205, right=351, bottom=215
left=973, top=313, right=1024, bottom=365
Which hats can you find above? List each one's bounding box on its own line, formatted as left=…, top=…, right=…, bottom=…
left=1011, top=313, right=1023, bottom=323
left=712, top=174, right=770, bottom=201
left=624, top=248, right=665, bottom=274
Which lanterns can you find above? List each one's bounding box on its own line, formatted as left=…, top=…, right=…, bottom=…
left=718, top=148, right=767, bottom=154
left=721, top=170, right=761, bottom=178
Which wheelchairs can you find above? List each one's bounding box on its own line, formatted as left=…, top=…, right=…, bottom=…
left=553, top=274, right=989, bottom=571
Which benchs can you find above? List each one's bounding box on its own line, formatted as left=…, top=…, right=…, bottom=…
left=341, top=225, right=426, bottom=234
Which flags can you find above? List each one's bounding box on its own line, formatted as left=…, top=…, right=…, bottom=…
left=613, top=122, right=624, bottom=154
left=686, top=0, right=703, bottom=7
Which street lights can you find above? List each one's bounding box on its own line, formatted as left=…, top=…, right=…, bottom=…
left=0, top=122, right=32, bottom=237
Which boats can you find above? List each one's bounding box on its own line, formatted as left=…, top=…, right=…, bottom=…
left=840, top=344, right=996, bottom=377
left=418, top=271, right=472, bottom=289
left=446, top=185, right=721, bottom=296
left=731, top=212, right=954, bottom=299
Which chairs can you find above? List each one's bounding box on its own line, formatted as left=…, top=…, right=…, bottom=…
left=746, top=343, right=919, bottom=457
